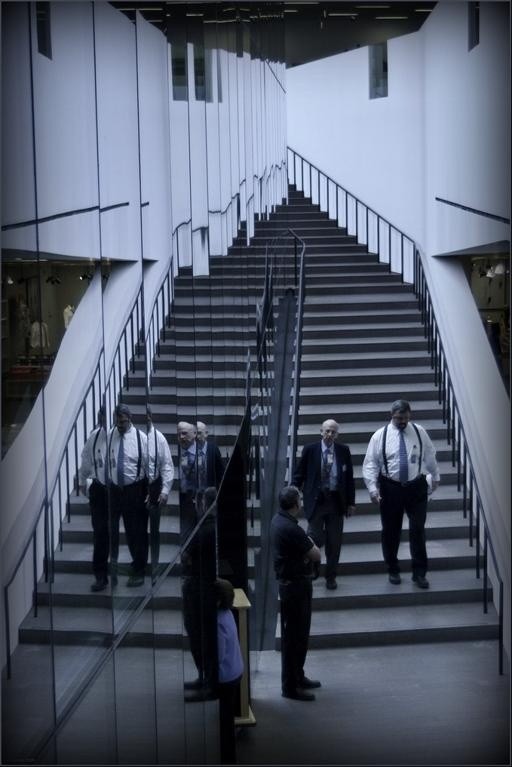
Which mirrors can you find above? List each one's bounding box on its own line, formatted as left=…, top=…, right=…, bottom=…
left=0, top=0, right=294, bottom=765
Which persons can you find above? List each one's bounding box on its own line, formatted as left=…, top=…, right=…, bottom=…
left=216, top=573, right=245, bottom=765
left=79, top=404, right=174, bottom=592
left=289, top=418, right=357, bottom=590
left=362, top=399, right=441, bottom=589
left=182, top=486, right=224, bottom=704
left=268, top=485, right=321, bottom=702
left=175, top=421, right=223, bottom=550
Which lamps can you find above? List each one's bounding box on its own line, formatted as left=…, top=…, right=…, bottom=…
left=485, top=264, right=496, bottom=278
left=494, top=257, right=510, bottom=274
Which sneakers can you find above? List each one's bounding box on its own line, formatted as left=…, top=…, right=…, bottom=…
left=185, top=681, right=203, bottom=689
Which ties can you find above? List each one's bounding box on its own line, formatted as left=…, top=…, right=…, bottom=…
left=399, top=430, right=408, bottom=487
left=117, top=435, right=124, bottom=490
left=322, top=449, right=330, bottom=497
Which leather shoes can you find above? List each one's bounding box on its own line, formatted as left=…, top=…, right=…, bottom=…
left=327, top=579, right=336, bottom=589
left=185, top=690, right=218, bottom=703
left=412, top=575, right=428, bottom=588
left=127, top=577, right=144, bottom=586
left=91, top=578, right=108, bottom=591
left=389, top=574, right=401, bottom=584
left=297, top=677, right=320, bottom=688
left=282, top=691, right=315, bottom=701
